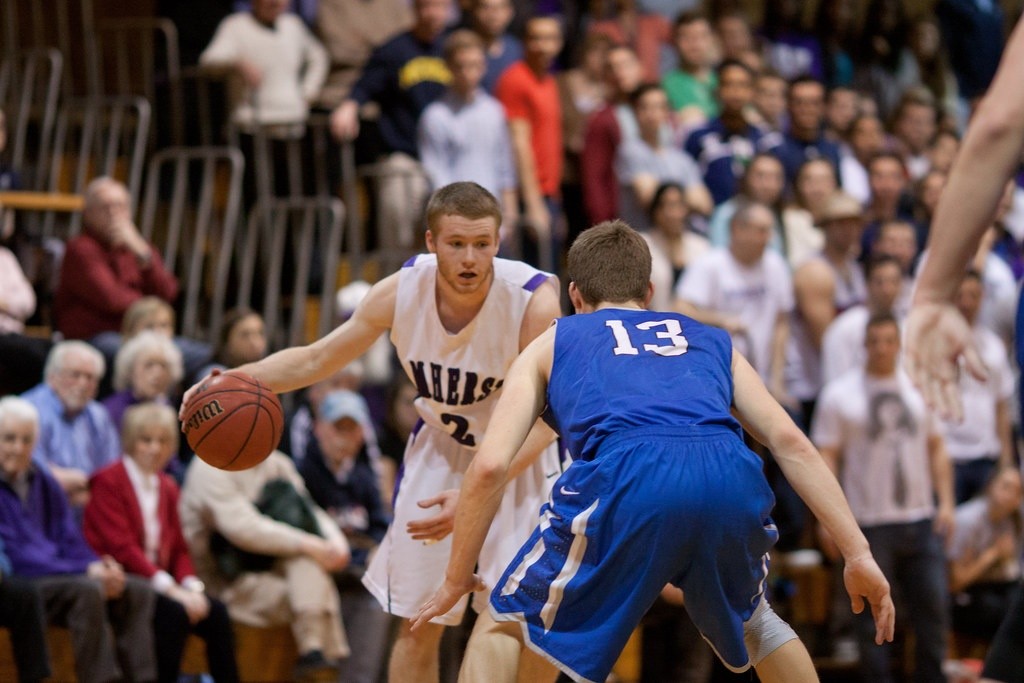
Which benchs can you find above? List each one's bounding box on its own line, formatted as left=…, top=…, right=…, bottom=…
left=0, top=118, right=372, bottom=682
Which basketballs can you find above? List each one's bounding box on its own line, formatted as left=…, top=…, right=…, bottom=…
left=183, top=369, right=284, bottom=475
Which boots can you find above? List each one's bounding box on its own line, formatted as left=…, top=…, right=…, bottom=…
left=289, top=610, right=352, bottom=668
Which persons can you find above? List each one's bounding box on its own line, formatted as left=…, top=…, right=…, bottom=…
left=198, top=0, right=329, bottom=136
left=331, top=0, right=1024, bottom=683
left=59, top=177, right=214, bottom=373
left=179, top=181, right=562, bottom=683
left=409, top=217, right=895, bottom=683
left=0, top=109, right=421, bottom=683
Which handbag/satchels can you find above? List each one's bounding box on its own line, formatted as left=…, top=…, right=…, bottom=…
left=208, top=479, right=326, bottom=584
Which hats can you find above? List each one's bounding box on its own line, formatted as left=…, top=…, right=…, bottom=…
left=320, top=389, right=370, bottom=427
left=333, top=280, right=372, bottom=318
left=813, top=193, right=863, bottom=221
